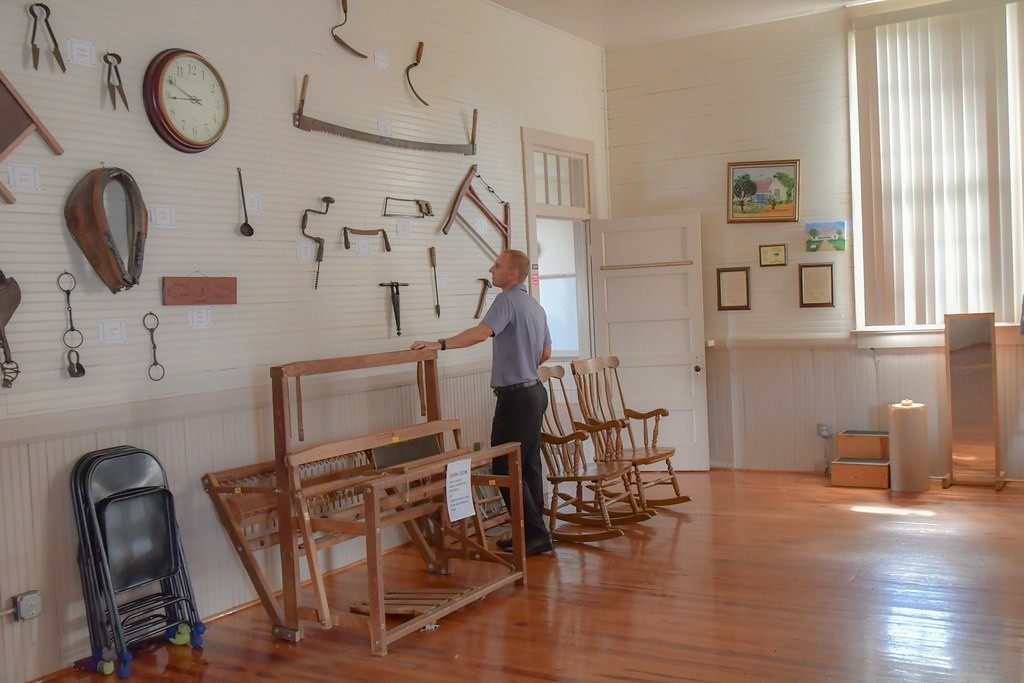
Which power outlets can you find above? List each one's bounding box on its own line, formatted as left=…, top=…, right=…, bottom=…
left=11, top=589, right=43, bottom=622
left=817, top=424, right=833, bottom=439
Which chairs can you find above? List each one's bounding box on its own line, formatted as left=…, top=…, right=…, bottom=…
left=538, top=364, right=652, bottom=544
left=558, top=356, right=693, bottom=516
left=70, top=443, right=205, bottom=680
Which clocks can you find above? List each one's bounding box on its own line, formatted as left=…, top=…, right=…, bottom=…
left=142, top=48, right=230, bottom=154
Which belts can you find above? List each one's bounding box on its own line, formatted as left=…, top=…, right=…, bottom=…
left=493, top=379, right=540, bottom=396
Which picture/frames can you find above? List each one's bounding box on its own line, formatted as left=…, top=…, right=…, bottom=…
left=716, top=266, right=751, bottom=311
left=798, top=262, right=836, bottom=308
left=759, top=243, right=788, bottom=267
left=727, top=158, right=799, bottom=224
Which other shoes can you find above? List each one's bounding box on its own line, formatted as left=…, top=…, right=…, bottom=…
left=503, top=535, right=553, bottom=556
left=496, top=538, right=512, bottom=548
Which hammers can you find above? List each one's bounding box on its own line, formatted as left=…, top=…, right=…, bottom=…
left=475, top=278, right=493, bottom=319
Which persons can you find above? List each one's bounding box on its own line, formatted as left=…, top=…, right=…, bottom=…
left=409, top=249, right=552, bottom=556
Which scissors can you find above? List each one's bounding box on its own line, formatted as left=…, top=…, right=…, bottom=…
left=29, top=3, right=66, bottom=73
left=104, top=52, right=130, bottom=113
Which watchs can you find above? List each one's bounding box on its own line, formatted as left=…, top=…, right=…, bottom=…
left=438, top=338, right=446, bottom=350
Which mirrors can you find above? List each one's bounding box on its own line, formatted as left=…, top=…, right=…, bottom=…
left=944, top=312, right=1006, bottom=491
